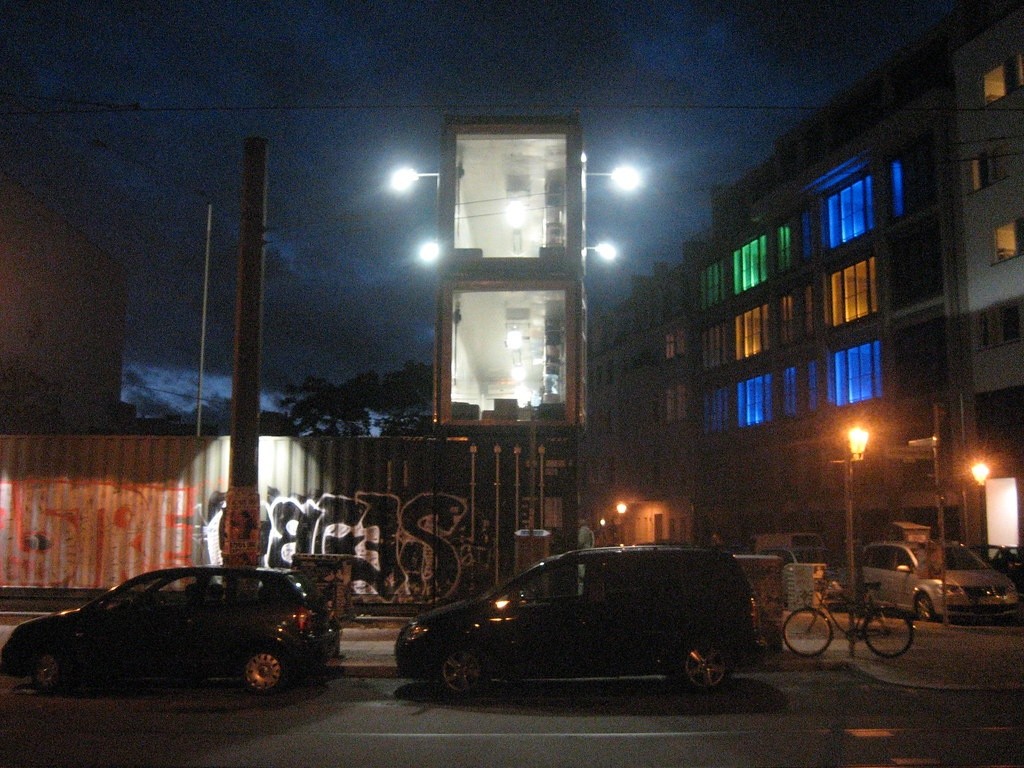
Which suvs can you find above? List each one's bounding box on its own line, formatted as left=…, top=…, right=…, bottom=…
left=392, top=543, right=768, bottom=696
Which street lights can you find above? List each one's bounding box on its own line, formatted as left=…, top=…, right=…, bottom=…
left=960, top=462, right=989, bottom=547
left=840, top=426, right=871, bottom=597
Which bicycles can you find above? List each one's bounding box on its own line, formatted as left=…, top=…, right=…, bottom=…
left=783, top=574, right=918, bottom=659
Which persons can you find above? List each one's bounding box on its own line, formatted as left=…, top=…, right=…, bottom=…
left=575, top=520, right=594, bottom=592
left=837, top=535, right=868, bottom=621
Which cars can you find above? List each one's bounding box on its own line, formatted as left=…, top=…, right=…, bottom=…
left=861, top=534, right=1019, bottom=623
left=985, top=548, right=1024, bottom=591
left=0, top=565, right=346, bottom=708
left=742, top=532, right=839, bottom=587
